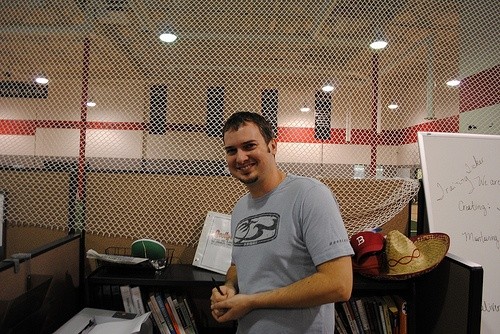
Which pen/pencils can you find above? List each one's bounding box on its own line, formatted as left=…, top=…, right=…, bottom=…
left=210, top=276, right=224, bottom=296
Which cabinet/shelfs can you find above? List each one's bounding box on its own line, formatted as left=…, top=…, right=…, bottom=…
left=86, top=261, right=426, bottom=334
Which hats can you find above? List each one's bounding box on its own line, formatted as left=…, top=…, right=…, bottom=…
left=384, top=230, right=450, bottom=280
left=349, top=232, right=385, bottom=262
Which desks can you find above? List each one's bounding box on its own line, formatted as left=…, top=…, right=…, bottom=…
left=44, top=306, right=155, bottom=334
left=0, top=267, right=54, bottom=334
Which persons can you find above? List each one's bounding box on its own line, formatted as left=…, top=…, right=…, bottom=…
left=210, top=111, right=355, bottom=334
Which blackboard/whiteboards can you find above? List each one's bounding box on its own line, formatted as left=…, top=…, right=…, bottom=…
left=416, top=132, right=500, bottom=334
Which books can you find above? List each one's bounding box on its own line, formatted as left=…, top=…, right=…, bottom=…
left=334, top=294, right=408, bottom=334
left=120, top=285, right=204, bottom=334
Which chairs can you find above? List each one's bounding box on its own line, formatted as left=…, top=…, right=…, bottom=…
left=130, top=240, right=167, bottom=268
